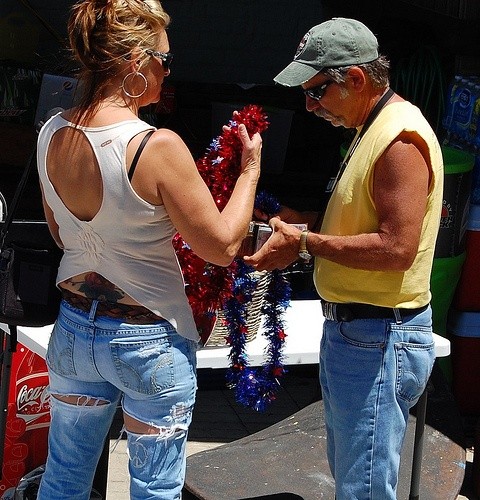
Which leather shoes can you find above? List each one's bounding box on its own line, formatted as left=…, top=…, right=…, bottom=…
left=59, top=285, right=167, bottom=324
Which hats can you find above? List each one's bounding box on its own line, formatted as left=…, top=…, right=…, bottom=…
left=274, top=18, right=379, bottom=87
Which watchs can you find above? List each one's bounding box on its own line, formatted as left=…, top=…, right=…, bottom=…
left=298, top=234, right=312, bottom=263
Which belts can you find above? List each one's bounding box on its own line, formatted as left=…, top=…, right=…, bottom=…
left=321, top=299, right=429, bottom=322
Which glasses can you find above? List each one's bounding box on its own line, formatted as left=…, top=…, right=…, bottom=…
left=123, top=49, right=176, bottom=69
left=304, top=72, right=346, bottom=101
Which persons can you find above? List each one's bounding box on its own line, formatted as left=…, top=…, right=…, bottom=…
left=243, top=17, right=444, bottom=500
left=139, top=71, right=205, bottom=158
left=36, top=0, right=264, bottom=500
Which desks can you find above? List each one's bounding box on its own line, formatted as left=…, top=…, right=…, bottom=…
left=0, top=300, right=452, bottom=500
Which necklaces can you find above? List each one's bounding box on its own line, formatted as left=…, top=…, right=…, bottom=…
left=172, top=105, right=291, bottom=411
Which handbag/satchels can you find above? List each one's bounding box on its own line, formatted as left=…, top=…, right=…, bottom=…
left=0, top=107, right=65, bottom=327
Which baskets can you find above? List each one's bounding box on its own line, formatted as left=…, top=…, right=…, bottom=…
left=184, top=270, right=273, bottom=347
